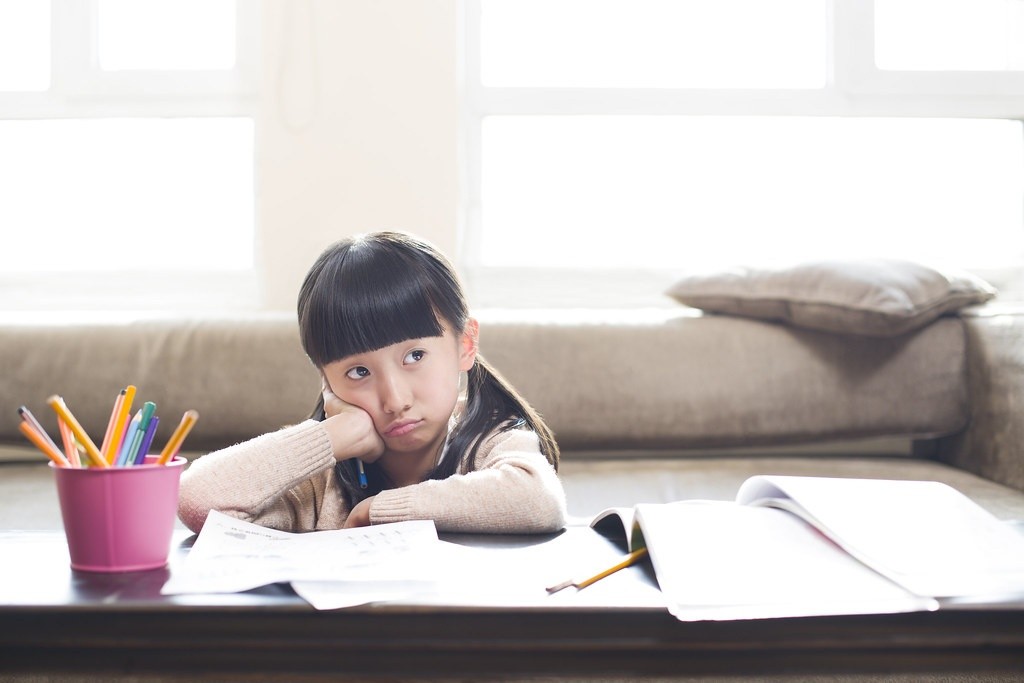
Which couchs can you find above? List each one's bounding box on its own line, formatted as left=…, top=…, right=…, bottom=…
left=0, top=313, right=1024, bottom=683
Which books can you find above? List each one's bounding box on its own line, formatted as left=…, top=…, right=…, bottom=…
left=590, top=475, right=1024, bottom=621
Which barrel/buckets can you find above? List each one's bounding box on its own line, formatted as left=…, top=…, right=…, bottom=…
left=49, top=455, right=187, bottom=572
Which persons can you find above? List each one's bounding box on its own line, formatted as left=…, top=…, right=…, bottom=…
left=178, top=232, right=567, bottom=534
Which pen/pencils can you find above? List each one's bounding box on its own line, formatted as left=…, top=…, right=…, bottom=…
left=17, top=385, right=199, bottom=468
left=545, top=547, right=649, bottom=594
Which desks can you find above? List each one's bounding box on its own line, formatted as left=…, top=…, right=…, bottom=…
left=1, top=533, right=1023, bottom=683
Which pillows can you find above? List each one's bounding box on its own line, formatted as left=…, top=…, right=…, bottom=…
left=666, top=256, right=999, bottom=337
